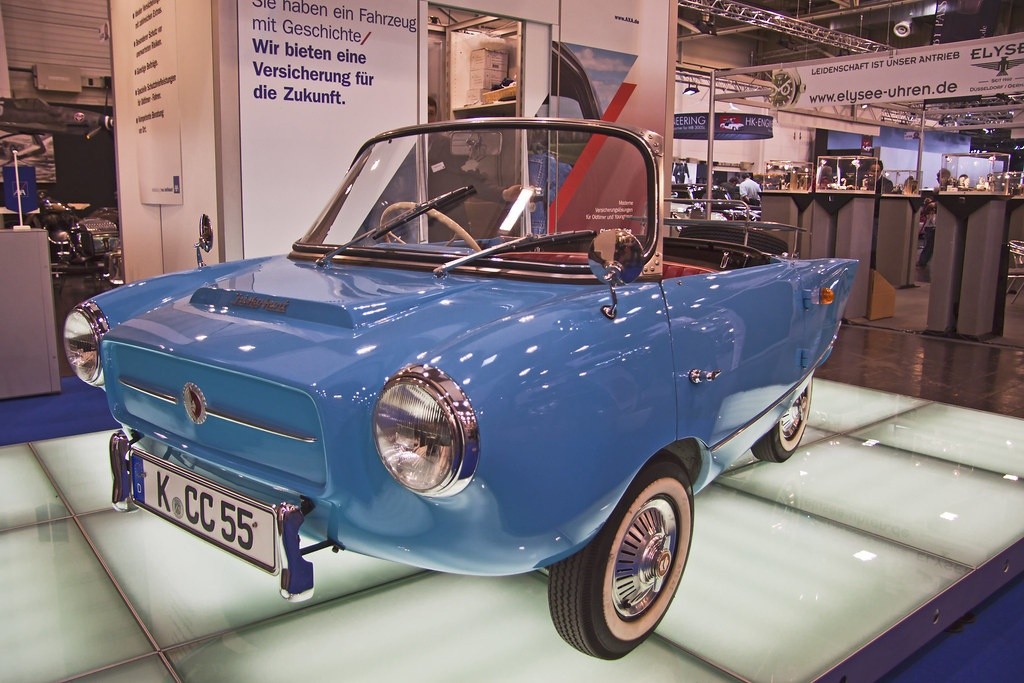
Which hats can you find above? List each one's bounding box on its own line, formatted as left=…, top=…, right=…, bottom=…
left=740, top=173, right=750, bottom=179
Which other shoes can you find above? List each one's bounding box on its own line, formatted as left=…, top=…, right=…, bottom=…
left=915, top=260, right=926, bottom=268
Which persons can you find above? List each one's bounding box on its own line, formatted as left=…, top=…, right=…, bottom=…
left=808, top=166, right=833, bottom=192
left=723, top=177, right=738, bottom=200
left=739, top=173, right=762, bottom=207
left=868, top=161, right=893, bottom=192
left=842, top=164, right=862, bottom=186
left=426, top=96, right=523, bottom=243
left=903, top=169, right=968, bottom=267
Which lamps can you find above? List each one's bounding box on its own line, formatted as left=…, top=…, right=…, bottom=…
left=682, top=82, right=700, bottom=96
left=776, top=33, right=799, bottom=51
left=694, top=12, right=717, bottom=37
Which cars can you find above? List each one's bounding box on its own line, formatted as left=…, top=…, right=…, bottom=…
left=670, top=184, right=761, bottom=222
left=64, top=116, right=860, bottom=661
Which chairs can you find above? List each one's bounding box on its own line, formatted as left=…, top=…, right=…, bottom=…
left=1007, top=240, right=1024, bottom=305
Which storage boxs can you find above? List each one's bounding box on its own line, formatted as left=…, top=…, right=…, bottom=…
left=470, top=48, right=509, bottom=71
left=469, top=69, right=507, bottom=89
left=466, top=89, right=491, bottom=104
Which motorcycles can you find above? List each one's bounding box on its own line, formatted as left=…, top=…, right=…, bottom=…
left=6, top=190, right=118, bottom=278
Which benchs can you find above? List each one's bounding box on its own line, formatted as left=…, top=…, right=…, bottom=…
left=517, top=236, right=770, bottom=270
